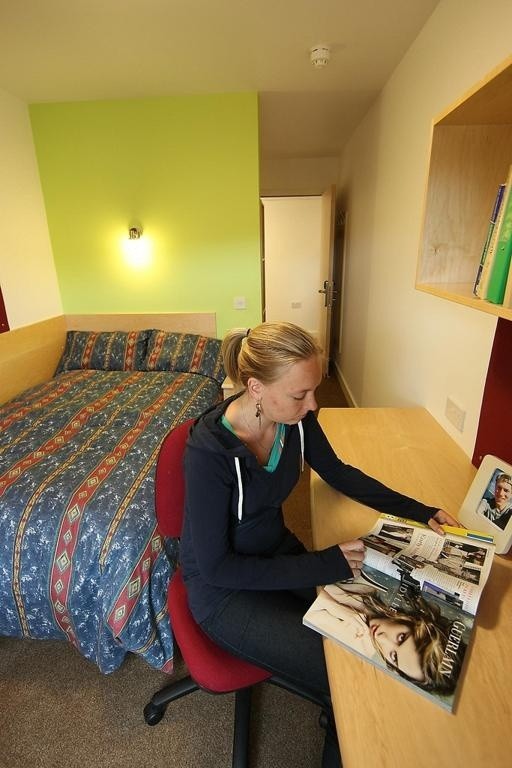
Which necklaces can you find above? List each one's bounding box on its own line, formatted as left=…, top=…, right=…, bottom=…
left=240, top=400, right=276, bottom=454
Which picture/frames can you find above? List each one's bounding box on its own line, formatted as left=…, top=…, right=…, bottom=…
left=457, top=455, right=512, bottom=556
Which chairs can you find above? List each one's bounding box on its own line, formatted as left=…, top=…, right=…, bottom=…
left=142, top=415, right=332, bottom=768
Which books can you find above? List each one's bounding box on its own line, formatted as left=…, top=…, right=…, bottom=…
left=303, top=512, right=494, bottom=714
left=472, top=166, right=512, bottom=310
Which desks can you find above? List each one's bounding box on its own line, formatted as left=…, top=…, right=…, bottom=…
left=312, top=406, right=510, bottom=768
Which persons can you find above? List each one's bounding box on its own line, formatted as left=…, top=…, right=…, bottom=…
left=303, top=572, right=466, bottom=697
left=180, top=321, right=459, bottom=699
left=443, top=541, right=486, bottom=559
left=478, top=473, right=512, bottom=531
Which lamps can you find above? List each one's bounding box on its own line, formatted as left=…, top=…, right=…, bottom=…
left=309, top=45, right=335, bottom=73
left=122, top=223, right=149, bottom=263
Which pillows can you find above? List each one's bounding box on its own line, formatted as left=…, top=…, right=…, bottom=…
left=55, top=325, right=153, bottom=372
left=138, top=330, right=228, bottom=385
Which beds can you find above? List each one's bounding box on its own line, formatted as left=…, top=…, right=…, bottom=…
left=0, top=314, right=216, bottom=673
